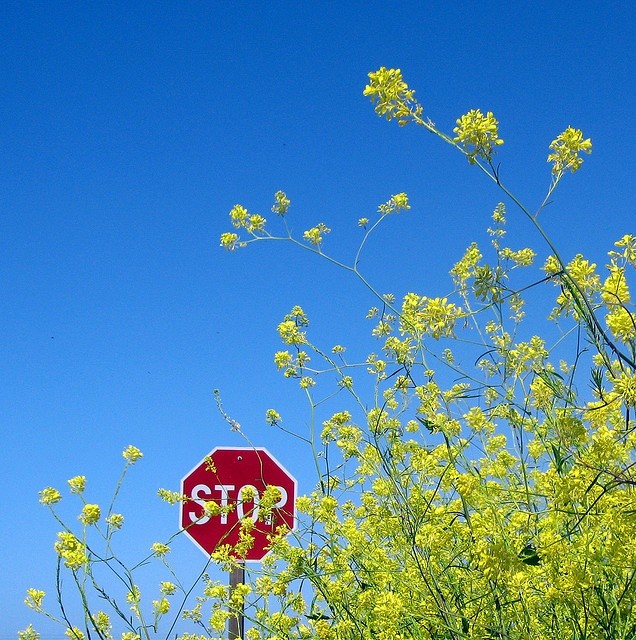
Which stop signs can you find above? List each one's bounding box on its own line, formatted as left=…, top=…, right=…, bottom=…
left=179, top=447, right=297, bottom=564
